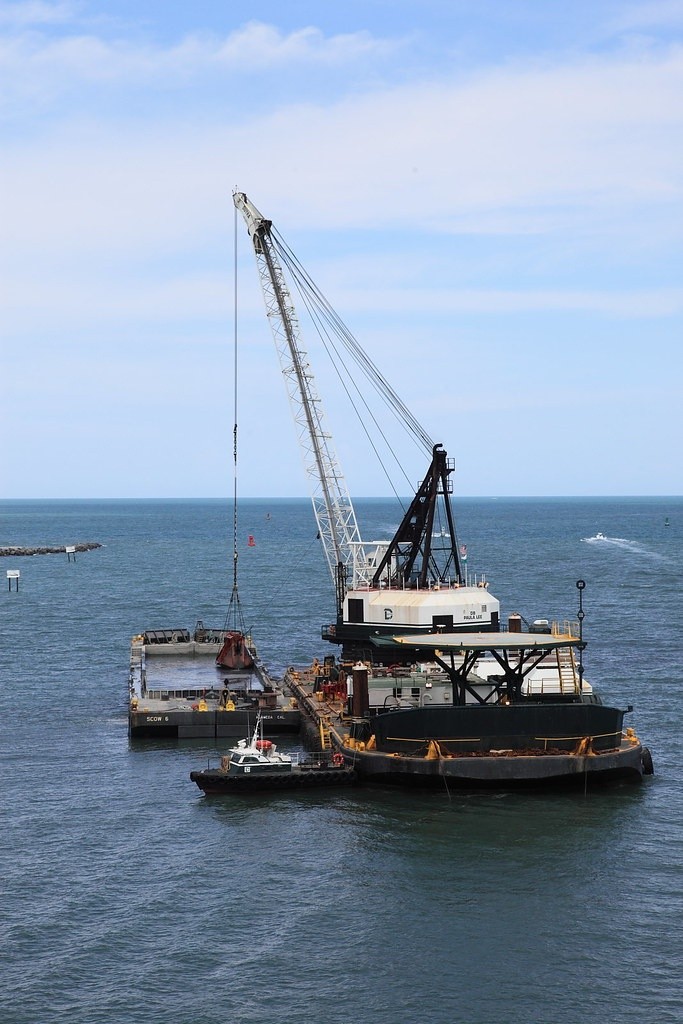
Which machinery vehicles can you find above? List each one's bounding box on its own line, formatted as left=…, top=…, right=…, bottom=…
left=230, top=190, right=501, bottom=635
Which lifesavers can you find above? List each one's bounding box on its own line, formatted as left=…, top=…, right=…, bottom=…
left=333, top=754, right=344, bottom=765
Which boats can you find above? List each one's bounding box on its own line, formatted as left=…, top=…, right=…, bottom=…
left=189, top=698, right=361, bottom=796
left=127, top=619, right=304, bottom=741
left=279, top=656, right=655, bottom=796
